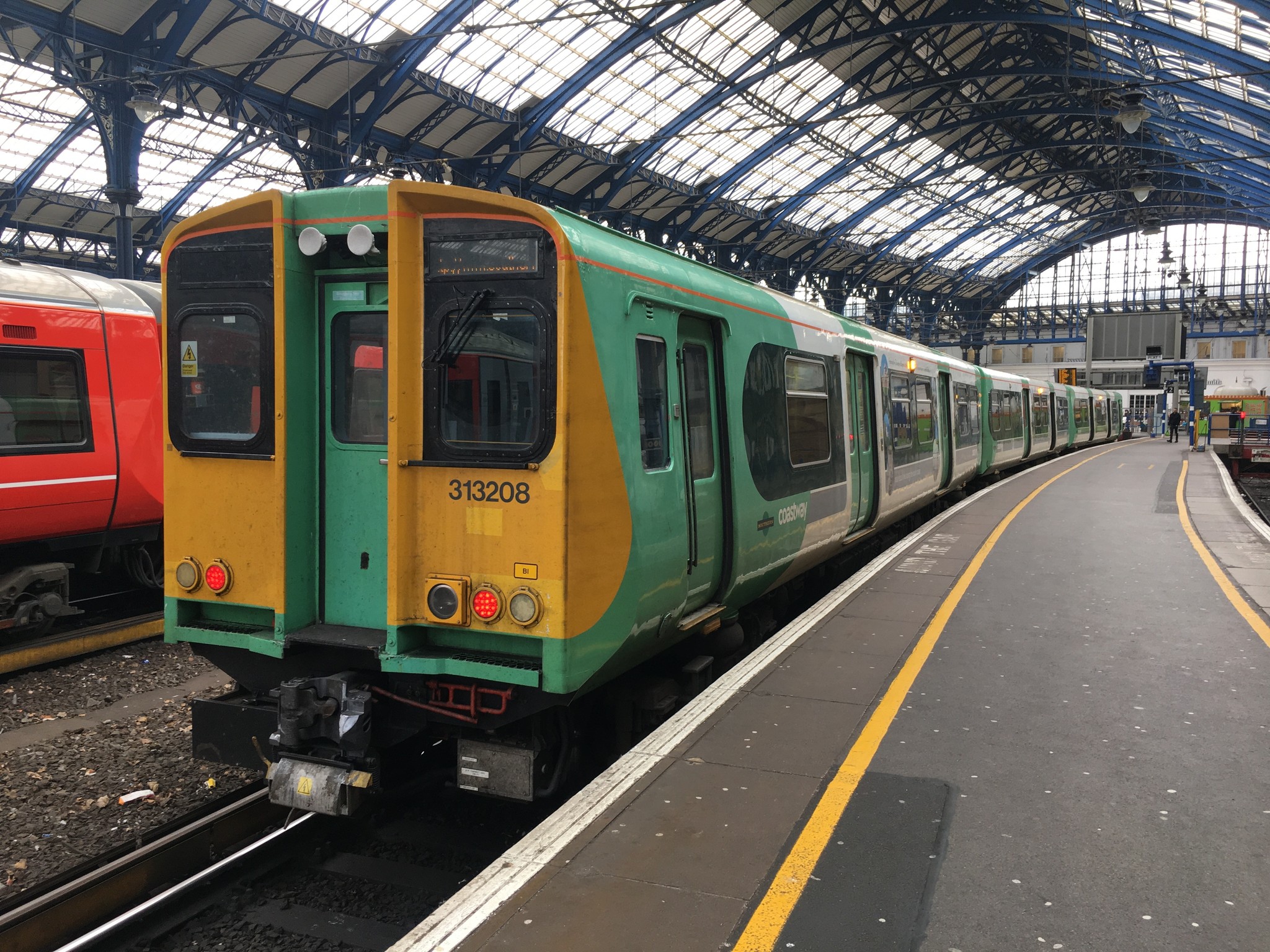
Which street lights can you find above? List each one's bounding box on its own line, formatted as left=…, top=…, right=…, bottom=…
left=1023, top=269, right=1038, bottom=339
left=1075, top=239, right=1091, bottom=338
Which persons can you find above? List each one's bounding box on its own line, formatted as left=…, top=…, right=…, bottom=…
left=1123, top=410, right=1131, bottom=427
left=1167, top=408, right=1181, bottom=443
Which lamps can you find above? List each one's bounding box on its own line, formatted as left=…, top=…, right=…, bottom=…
left=1142, top=209, right=1163, bottom=236
left=1111, top=81, right=1152, bottom=134
left=1128, top=161, right=1156, bottom=202
left=1159, top=242, right=1176, bottom=270
left=1213, top=299, right=1227, bottom=316
left=1259, top=325, right=1267, bottom=338
left=1176, top=265, right=1193, bottom=291
left=124, top=67, right=165, bottom=124
left=1237, top=310, right=1248, bottom=333
left=1195, top=285, right=1208, bottom=303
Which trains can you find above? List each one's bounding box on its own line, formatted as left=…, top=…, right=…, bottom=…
left=1, top=254, right=162, bottom=652
left=164, top=176, right=1125, bottom=819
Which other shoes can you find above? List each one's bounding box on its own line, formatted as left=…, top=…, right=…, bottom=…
left=1167, top=439, right=1172, bottom=443
left=1175, top=441, right=1178, bottom=443
